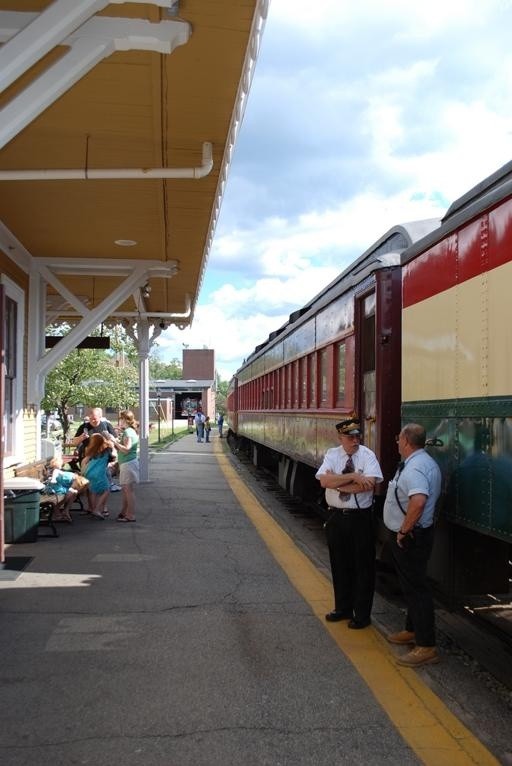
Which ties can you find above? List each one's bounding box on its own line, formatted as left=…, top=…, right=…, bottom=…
left=339, top=455, right=354, bottom=501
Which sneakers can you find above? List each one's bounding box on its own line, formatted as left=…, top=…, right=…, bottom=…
left=109, top=484, right=121, bottom=491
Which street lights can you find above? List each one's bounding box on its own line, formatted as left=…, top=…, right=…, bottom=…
left=155, top=388, right=161, bottom=441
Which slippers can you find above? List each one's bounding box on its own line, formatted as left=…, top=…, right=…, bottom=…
left=80, top=509, right=136, bottom=522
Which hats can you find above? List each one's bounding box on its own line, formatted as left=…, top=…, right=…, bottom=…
left=336, top=419, right=361, bottom=434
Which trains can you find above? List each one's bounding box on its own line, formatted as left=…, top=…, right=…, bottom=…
left=225, top=157, right=511, bottom=636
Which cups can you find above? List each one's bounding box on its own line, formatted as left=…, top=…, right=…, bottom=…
left=83, top=428, right=88, bottom=435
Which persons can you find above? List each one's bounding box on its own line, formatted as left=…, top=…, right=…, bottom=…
left=314, top=419, right=384, bottom=629
left=205, top=417, right=211, bottom=442
left=218, top=414, right=224, bottom=438
left=39, top=406, right=155, bottom=524
left=382, top=423, right=442, bottom=666
left=194, top=407, right=206, bottom=438
left=195, top=414, right=203, bottom=443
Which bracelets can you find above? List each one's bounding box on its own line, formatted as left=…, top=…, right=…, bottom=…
left=399, top=530, right=408, bottom=535
left=336, top=487, right=339, bottom=491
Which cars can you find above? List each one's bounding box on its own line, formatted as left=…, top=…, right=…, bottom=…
left=40, top=410, right=62, bottom=431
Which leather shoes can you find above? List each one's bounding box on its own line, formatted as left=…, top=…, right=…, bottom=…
left=397, top=647, right=440, bottom=667
left=326, top=610, right=371, bottom=628
left=388, top=631, right=416, bottom=644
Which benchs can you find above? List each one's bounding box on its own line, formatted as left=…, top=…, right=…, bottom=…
left=14, top=454, right=94, bottom=539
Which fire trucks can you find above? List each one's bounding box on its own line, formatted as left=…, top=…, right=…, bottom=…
left=180, top=398, right=202, bottom=417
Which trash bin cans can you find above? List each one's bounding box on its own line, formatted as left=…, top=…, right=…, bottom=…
left=4, top=476, right=45, bottom=543
